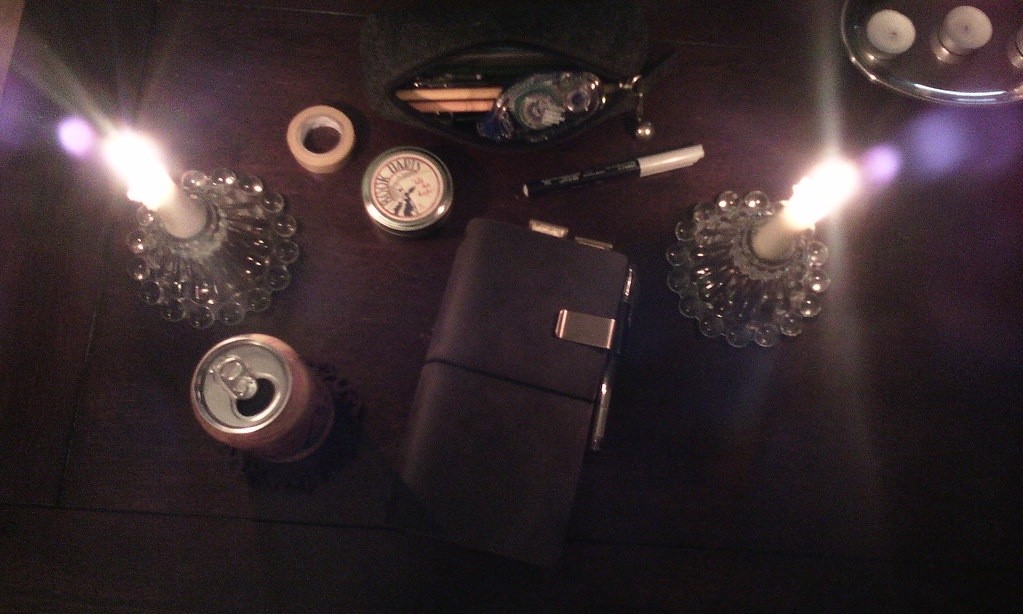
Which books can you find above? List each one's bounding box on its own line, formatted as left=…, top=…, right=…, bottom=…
left=391, top=217, right=629, bottom=566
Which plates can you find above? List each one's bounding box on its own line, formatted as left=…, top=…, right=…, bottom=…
left=838, top=1, right=1023, bottom=108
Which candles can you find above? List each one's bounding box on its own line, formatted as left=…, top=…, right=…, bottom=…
left=938, top=5, right=994, bottom=57
left=864, top=9, right=917, bottom=60
left=106, top=138, right=201, bottom=236
left=752, top=169, right=839, bottom=258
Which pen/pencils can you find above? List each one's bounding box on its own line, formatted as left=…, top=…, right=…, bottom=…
left=523, top=145, right=705, bottom=197
left=588, top=267, right=638, bottom=453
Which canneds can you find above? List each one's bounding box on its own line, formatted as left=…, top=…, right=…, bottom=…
left=192, top=334, right=336, bottom=464
